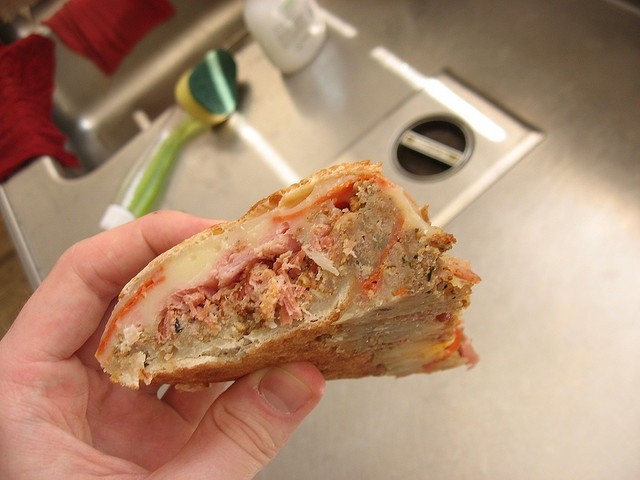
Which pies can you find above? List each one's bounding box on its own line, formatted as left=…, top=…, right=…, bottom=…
left=92, top=160, right=483, bottom=388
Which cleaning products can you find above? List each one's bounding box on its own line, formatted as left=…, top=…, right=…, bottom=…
left=243, top=0, right=328, bottom=76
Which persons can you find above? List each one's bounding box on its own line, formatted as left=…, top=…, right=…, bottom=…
left=1, top=210, right=326, bottom=480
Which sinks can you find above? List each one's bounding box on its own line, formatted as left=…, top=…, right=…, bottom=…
left=0, top=0, right=258, bottom=185
